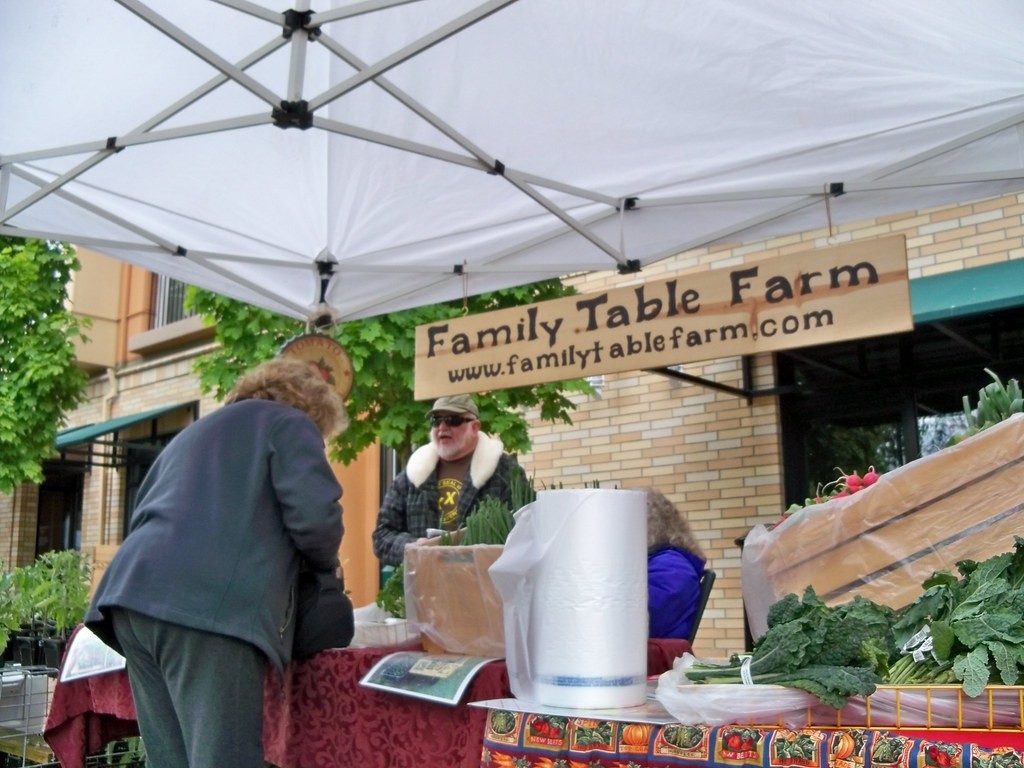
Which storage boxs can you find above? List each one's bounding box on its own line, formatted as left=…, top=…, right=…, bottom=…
left=404, top=510, right=505, bottom=659
left=762, top=413, right=1024, bottom=611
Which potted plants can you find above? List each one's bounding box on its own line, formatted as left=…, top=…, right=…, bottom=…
left=0, top=549, right=91, bottom=678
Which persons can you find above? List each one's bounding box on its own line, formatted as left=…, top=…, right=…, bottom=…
left=84, top=355, right=349, bottom=768
left=632, top=486, right=707, bottom=677
left=372, top=391, right=536, bottom=568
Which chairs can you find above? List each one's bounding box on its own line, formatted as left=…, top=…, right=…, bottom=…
left=687, top=568, right=716, bottom=646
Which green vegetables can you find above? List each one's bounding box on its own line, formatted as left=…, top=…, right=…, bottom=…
left=375, top=563, right=405, bottom=618
left=686, top=535, right=1024, bottom=710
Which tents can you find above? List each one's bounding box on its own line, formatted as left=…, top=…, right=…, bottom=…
left=0, top=0, right=1024, bottom=333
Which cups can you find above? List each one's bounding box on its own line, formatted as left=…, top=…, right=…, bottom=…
left=426, top=528, right=445, bottom=538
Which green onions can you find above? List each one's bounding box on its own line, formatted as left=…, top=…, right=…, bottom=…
left=439, top=467, right=618, bottom=546
left=962, top=368, right=1024, bottom=436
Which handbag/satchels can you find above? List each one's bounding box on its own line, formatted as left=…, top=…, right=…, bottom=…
left=292, top=561, right=355, bottom=659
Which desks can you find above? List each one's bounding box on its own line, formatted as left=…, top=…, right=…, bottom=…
left=41, top=638, right=694, bottom=768
left=478, top=676, right=1024, bottom=768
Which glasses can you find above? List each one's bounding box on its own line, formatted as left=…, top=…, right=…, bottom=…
left=430, top=415, right=475, bottom=427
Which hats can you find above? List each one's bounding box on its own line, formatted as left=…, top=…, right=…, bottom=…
left=424, top=394, right=479, bottom=418
